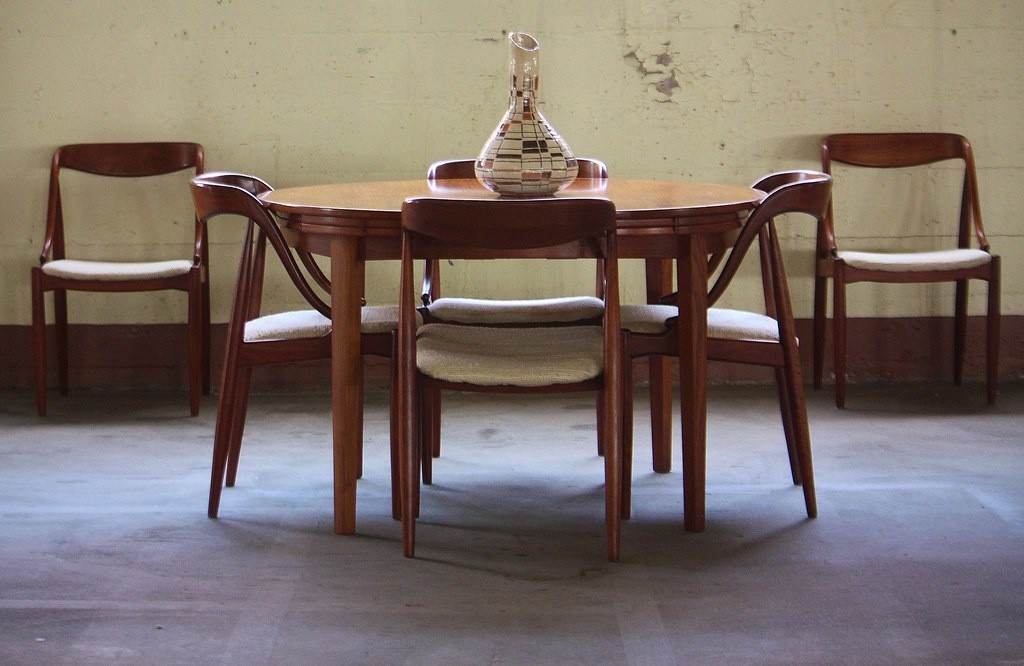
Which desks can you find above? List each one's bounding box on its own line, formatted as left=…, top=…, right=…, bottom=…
left=258, top=177, right=770, bottom=538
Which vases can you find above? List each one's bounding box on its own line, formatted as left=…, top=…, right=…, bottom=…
left=474, top=31, right=580, bottom=195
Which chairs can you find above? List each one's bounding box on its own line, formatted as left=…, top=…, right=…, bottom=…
left=421, top=157, right=610, bottom=488
left=619, top=169, right=835, bottom=535
left=191, top=171, right=425, bottom=523
left=30, top=141, right=210, bottom=420
left=397, top=195, right=621, bottom=564
left=811, top=128, right=1003, bottom=408
